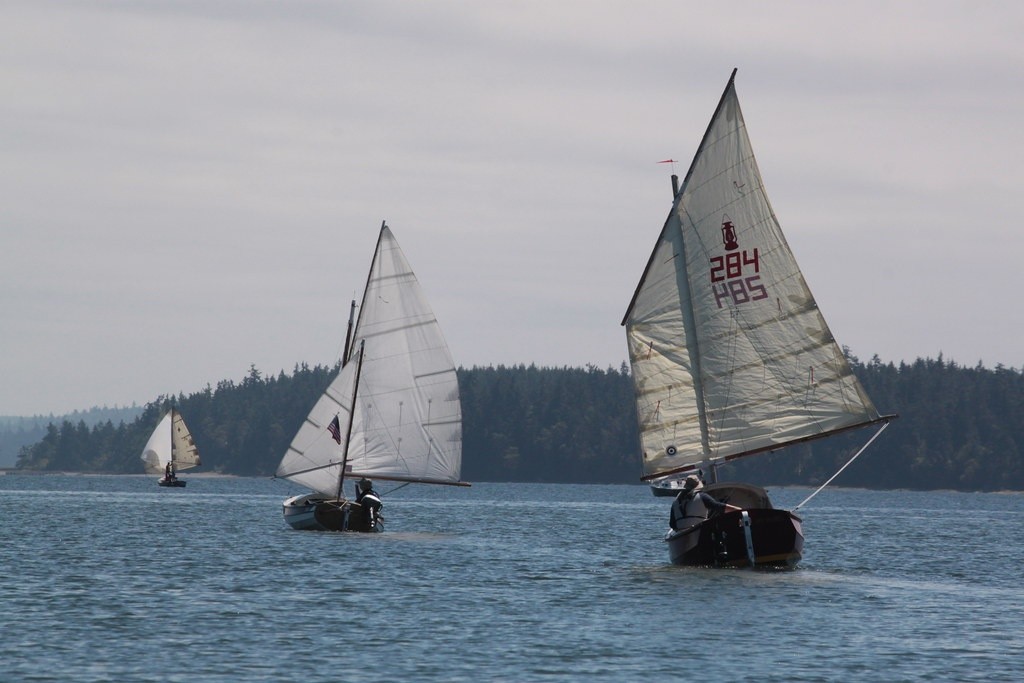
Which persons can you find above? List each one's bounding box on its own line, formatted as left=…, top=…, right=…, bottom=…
left=669, top=475, right=741, bottom=533
left=355, top=478, right=380, bottom=503
left=166, top=462, right=173, bottom=479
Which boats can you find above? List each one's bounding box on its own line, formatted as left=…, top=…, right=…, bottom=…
left=648, top=476, right=706, bottom=497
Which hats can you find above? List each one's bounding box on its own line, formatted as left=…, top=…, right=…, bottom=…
left=354, top=478, right=372, bottom=488
left=684, top=475, right=703, bottom=490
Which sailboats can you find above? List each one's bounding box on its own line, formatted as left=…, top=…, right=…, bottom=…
left=269, top=219, right=474, bottom=531
left=140, top=406, right=202, bottom=487
left=620, top=66, right=900, bottom=573
